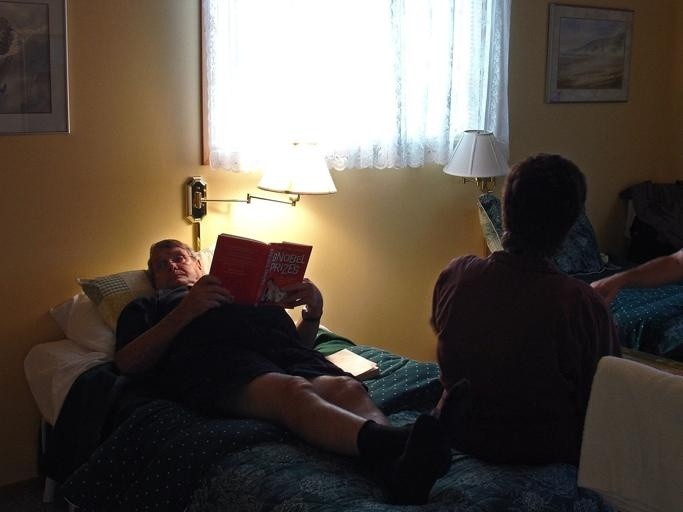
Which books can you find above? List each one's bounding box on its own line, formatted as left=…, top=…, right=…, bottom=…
left=208, top=234, right=313, bottom=309
left=325, top=349, right=381, bottom=381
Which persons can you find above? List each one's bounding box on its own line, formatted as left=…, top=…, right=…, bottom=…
left=114, top=240, right=454, bottom=505
left=429, top=153, right=622, bottom=466
left=589, top=247, right=683, bottom=305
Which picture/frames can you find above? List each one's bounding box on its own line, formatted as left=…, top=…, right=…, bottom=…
left=0, top=0, right=73, bottom=135
left=545, top=2, right=636, bottom=106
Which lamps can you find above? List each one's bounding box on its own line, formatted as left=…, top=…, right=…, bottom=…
left=442, top=130, right=507, bottom=259
left=185, top=145, right=338, bottom=250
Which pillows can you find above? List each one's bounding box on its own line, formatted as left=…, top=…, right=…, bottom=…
left=78, top=270, right=160, bottom=331
left=476, top=196, right=607, bottom=278
left=48, top=292, right=133, bottom=358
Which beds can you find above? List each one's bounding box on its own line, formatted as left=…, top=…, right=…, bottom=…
left=23, top=326, right=683, bottom=512
